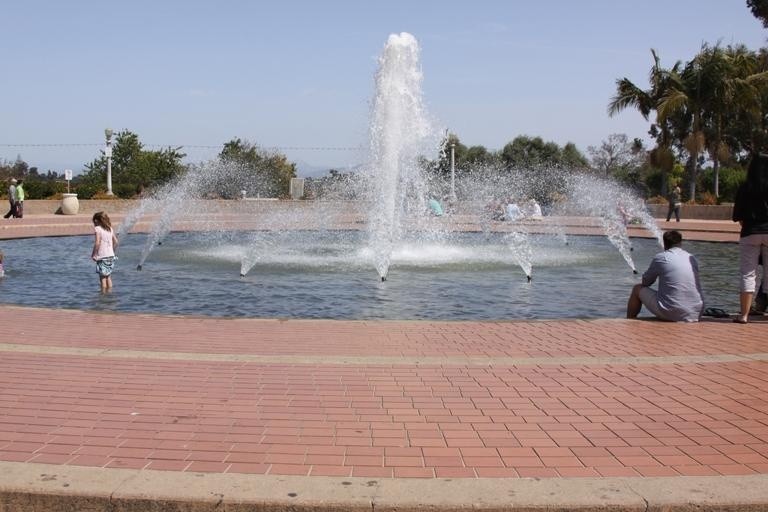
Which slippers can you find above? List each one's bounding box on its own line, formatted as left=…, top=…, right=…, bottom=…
left=702, top=307, right=730, bottom=316
left=733, top=316, right=747, bottom=323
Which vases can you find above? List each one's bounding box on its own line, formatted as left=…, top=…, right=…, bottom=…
left=61, top=192, right=79, bottom=215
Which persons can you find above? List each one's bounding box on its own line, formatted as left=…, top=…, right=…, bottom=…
left=628, top=231, right=704, bottom=323
left=15, top=180, right=24, bottom=218
left=617, top=203, right=630, bottom=226
left=485, top=198, right=541, bottom=223
left=91, top=212, right=118, bottom=292
left=733, top=152, right=768, bottom=323
left=4, top=178, right=17, bottom=218
left=428, top=198, right=442, bottom=216
left=666, top=184, right=681, bottom=221
